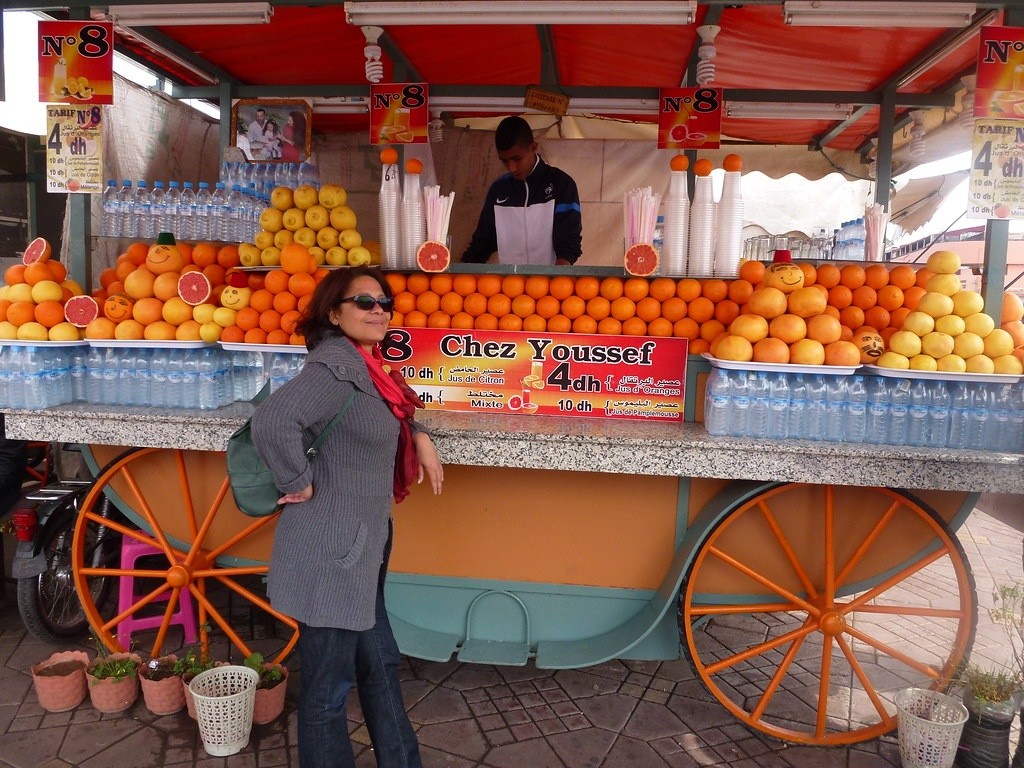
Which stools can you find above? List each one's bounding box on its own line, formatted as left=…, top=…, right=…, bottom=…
left=118, top=529, right=197, bottom=653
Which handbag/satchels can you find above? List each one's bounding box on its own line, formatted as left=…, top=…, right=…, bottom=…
left=226, top=389, right=355, bottom=517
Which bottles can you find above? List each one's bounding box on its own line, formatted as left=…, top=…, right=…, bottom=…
left=817, top=216, right=867, bottom=261
left=102, top=163, right=320, bottom=243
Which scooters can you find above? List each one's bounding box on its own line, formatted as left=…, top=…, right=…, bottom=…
left=12, top=442, right=119, bottom=646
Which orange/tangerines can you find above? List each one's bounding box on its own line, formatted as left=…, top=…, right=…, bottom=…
left=670, top=125, right=707, bottom=145
left=670, top=155, right=690, bottom=172
left=723, top=154, right=742, bottom=171
left=406, top=159, right=423, bottom=174
left=693, top=159, right=713, bottom=177
left=383, top=125, right=414, bottom=142
left=380, top=148, right=398, bottom=164
left=386, top=242, right=1024, bottom=374
left=995, top=90, right=1024, bottom=120
left=0, top=238, right=369, bottom=345
left=67, top=76, right=92, bottom=98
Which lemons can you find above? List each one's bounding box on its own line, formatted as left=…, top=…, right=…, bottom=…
left=237, top=185, right=380, bottom=269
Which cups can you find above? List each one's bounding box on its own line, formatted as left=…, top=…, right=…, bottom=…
left=393, top=109, right=410, bottom=132
left=377, top=164, right=456, bottom=269
left=660, top=170, right=830, bottom=279
left=1012, top=63, right=1023, bottom=93
left=53, top=64, right=67, bottom=95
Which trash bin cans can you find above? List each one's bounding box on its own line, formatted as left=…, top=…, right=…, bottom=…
left=188, top=666, right=258, bottom=757
left=893, top=688, right=969, bottom=768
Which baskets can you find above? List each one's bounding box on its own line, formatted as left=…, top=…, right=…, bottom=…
left=188, top=666, right=260, bottom=757
left=894, top=688, right=969, bottom=768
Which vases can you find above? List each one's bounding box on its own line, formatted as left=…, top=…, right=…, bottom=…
left=32, top=651, right=89, bottom=713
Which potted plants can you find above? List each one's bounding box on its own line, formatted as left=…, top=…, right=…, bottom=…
left=83, top=623, right=141, bottom=713
left=181, top=621, right=231, bottom=720
left=244, top=651, right=289, bottom=725
left=138, top=641, right=188, bottom=715
left=938, top=579, right=1024, bottom=768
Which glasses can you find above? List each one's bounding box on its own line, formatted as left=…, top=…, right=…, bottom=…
left=340, top=294, right=394, bottom=312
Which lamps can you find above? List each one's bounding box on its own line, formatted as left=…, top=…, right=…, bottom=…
left=344, top=1, right=697, bottom=25
left=782, top=1, right=976, bottom=27
left=724, top=100, right=852, bottom=120
left=257, top=96, right=369, bottom=114
left=90, top=3, right=274, bottom=26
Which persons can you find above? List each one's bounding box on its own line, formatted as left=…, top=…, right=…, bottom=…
left=251, top=267, right=444, bottom=768
left=248, top=109, right=307, bottom=163
left=460, top=116, right=583, bottom=266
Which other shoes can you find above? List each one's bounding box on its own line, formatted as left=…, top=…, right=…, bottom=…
left=272, top=150, right=281, bottom=158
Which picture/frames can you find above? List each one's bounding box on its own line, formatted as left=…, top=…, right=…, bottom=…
left=231, top=99, right=312, bottom=165
